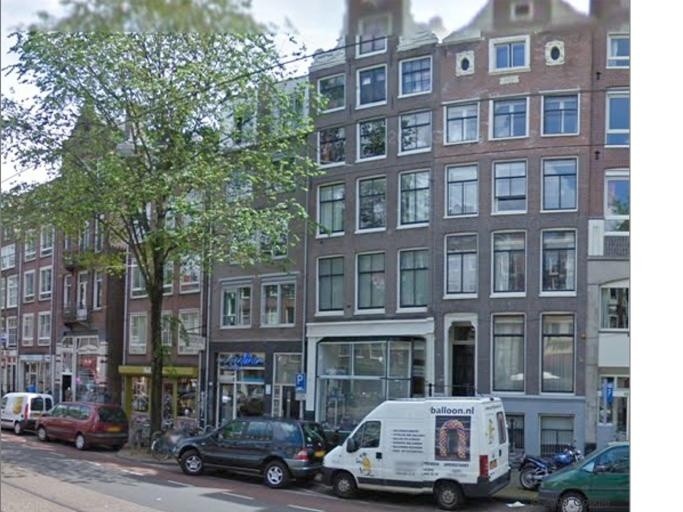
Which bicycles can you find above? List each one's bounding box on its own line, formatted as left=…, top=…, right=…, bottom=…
left=131, top=407, right=198, bottom=462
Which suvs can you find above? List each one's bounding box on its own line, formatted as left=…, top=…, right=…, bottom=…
left=172, top=414, right=329, bottom=490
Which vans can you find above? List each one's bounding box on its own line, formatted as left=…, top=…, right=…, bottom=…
left=35, top=398, right=129, bottom=452
left=320, top=395, right=513, bottom=512
left=0, top=391, right=55, bottom=435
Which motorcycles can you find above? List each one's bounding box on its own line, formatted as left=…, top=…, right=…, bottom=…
left=517, top=438, right=585, bottom=491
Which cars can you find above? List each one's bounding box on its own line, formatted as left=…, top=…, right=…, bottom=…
left=537, top=441, right=630, bottom=512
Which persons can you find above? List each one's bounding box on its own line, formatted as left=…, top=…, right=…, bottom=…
left=64, top=386, right=72, bottom=402
left=38, top=382, right=48, bottom=393
